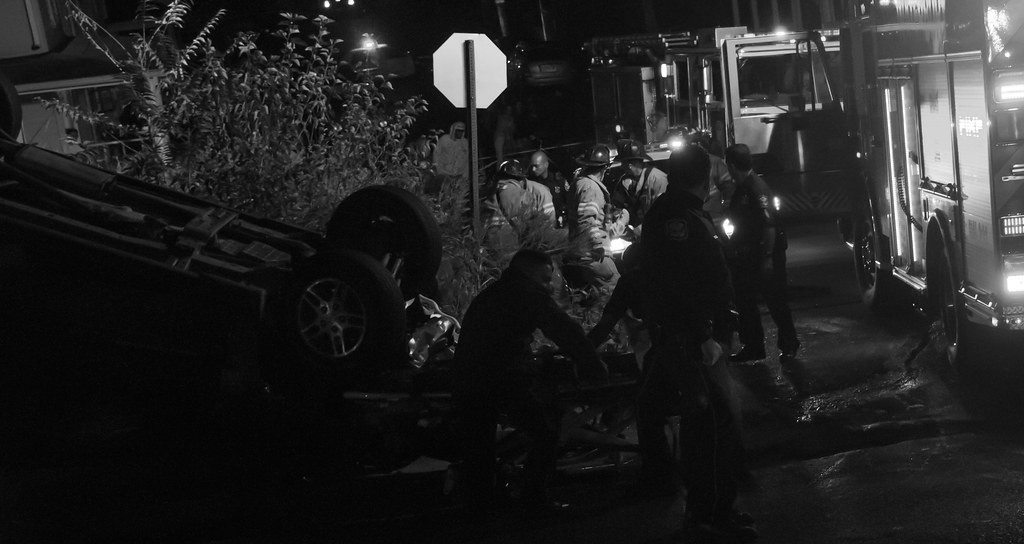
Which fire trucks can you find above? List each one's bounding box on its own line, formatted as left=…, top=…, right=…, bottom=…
left=581, top=0, right=1023, bottom=388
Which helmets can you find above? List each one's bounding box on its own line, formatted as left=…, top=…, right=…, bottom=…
left=613, top=140, right=653, bottom=162
left=499, top=158, right=522, bottom=177
left=575, top=144, right=609, bottom=167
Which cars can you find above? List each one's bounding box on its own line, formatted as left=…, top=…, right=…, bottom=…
left=0, top=75, right=468, bottom=419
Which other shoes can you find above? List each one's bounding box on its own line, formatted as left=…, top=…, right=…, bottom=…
left=686, top=512, right=757, bottom=541
left=779, top=341, right=800, bottom=361
left=531, top=499, right=571, bottom=514
left=730, top=348, right=766, bottom=363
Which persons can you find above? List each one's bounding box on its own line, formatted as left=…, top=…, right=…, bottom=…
left=403, top=103, right=801, bottom=362
left=641, top=146, right=759, bottom=541
left=586, top=240, right=754, bottom=500
left=453, top=246, right=610, bottom=476
